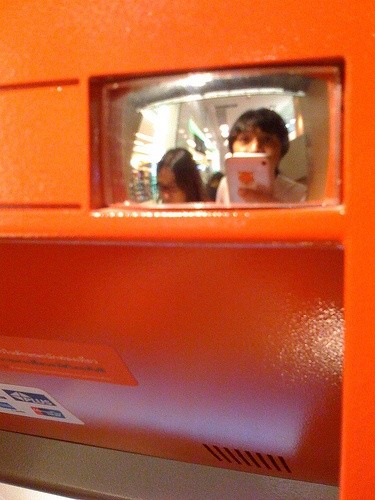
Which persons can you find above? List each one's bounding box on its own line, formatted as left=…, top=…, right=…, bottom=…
left=154, top=148, right=212, bottom=201
left=205, top=173, right=224, bottom=201
left=213, top=108, right=307, bottom=204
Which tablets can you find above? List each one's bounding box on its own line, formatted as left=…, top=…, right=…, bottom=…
left=225, top=152, right=273, bottom=204
